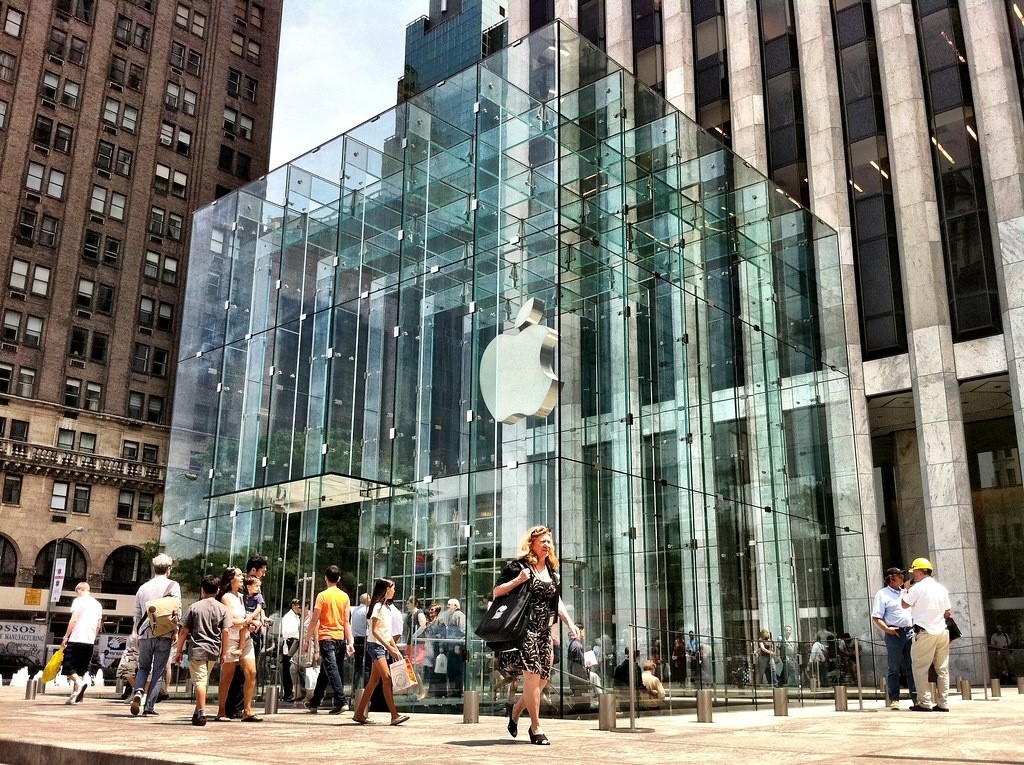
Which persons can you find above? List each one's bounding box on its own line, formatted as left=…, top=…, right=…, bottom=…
left=754, top=625, right=862, bottom=689
left=615, top=631, right=699, bottom=711
left=403, top=596, right=466, bottom=699
left=872, top=558, right=951, bottom=713
left=483, top=527, right=581, bottom=745
left=61, top=554, right=273, bottom=726
left=566, top=622, right=612, bottom=708
left=992, top=625, right=1011, bottom=655
left=283, top=565, right=410, bottom=727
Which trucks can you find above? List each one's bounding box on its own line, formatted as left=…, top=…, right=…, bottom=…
left=0, top=620, right=64, bottom=669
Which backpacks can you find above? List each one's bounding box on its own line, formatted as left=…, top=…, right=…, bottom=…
left=136, top=583, right=180, bottom=638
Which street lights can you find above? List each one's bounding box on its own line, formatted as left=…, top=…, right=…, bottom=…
left=42, top=526, right=85, bottom=672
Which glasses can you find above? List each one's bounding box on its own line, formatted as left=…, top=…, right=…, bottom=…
left=429, top=609, right=434, bottom=611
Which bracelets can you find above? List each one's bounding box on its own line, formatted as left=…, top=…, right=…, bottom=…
left=63, top=636, right=68, bottom=640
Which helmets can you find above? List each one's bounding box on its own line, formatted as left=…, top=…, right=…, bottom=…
left=909, top=556, right=934, bottom=575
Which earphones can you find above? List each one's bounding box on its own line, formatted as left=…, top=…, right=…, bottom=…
left=891, top=578, right=895, bottom=582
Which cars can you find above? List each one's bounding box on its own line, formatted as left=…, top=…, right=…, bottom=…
left=0, top=654, right=40, bottom=681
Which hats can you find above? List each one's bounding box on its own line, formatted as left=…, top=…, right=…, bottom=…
left=885, top=568, right=907, bottom=578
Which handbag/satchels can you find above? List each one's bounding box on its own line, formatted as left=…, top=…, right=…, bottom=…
left=567, top=641, right=587, bottom=689
left=305, top=667, right=320, bottom=690
left=434, top=652, right=448, bottom=674
left=477, top=562, right=532, bottom=650
left=41, top=645, right=65, bottom=682
left=944, top=617, right=963, bottom=644
left=583, top=650, right=598, bottom=669
left=389, top=654, right=418, bottom=694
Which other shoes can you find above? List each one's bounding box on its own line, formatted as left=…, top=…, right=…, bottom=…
left=909, top=702, right=950, bottom=713
left=120, top=683, right=409, bottom=726
left=64, top=682, right=88, bottom=705
left=417, top=690, right=429, bottom=701
left=891, top=699, right=899, bottom=709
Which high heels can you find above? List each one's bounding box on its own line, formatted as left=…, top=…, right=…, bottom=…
left=529, top=724, right=549, bottom=745
left=507, top=703, right=518, bottom=738
